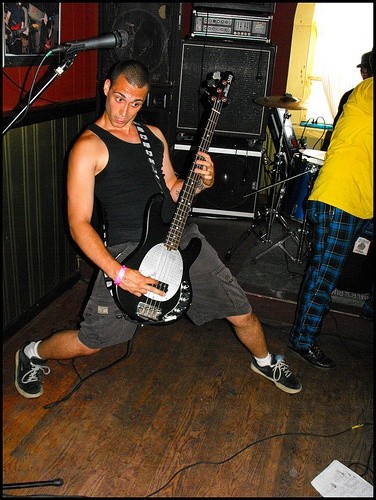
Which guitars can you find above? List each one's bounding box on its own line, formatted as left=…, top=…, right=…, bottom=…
left=112, top=71, right=235, bottom=327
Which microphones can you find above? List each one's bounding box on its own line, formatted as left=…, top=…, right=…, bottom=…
left=52, top=30, right=128, bottom=54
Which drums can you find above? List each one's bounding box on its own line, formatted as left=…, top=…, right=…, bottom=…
left=289, top=164, right=323, bottom=224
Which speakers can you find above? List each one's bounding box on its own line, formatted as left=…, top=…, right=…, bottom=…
left=97, top=2, right=184, bottom=88
left=176, top=39, right=277, bottom=141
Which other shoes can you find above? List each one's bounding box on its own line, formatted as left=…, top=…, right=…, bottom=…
left=15, top=340, right=45, bottom=399
left=250, top=354, right=302, bottom=394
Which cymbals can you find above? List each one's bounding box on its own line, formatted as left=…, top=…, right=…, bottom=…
left=300, top=157, right=327, bottom=168
left=255, top=93, right=303, bottom=111
left=300, top=149, right=331, bottom=160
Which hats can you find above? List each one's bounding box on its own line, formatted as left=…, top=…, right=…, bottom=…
left=357, top=52, right=372, bottom=68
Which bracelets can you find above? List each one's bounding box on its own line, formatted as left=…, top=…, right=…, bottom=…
left=114, top=264, right=127, bottom=286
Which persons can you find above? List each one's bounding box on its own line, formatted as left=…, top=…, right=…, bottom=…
left=333, top=50, right=372, bottom=129
left=3, top=1, right=57, bottom=56
left=284, top=50, right=375, bottom=371
left=14, top=60, right=303, bottom=399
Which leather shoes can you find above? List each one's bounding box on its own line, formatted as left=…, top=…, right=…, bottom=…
left=287, top=339, right=336, bottom=372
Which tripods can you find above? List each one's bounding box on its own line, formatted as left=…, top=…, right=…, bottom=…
left=223, top=107, right=313, bottom=262
